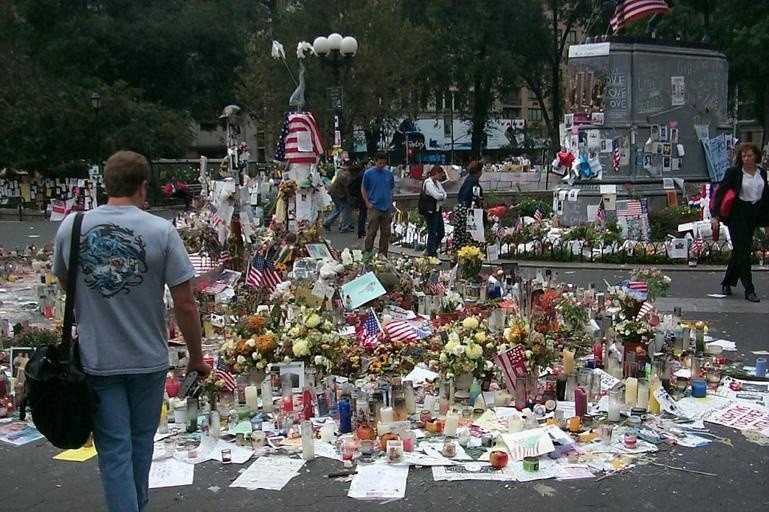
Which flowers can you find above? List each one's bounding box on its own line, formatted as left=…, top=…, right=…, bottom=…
left=190, top=245, right=672, bottom=399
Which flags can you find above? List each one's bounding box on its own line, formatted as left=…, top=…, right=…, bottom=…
left=248, top=254, right=266, bottom=288
left=610, top=1, right=669, bottom=39
left=362, top=307, right=431, bottom=346
left=261, top=260, right=282, bottom=289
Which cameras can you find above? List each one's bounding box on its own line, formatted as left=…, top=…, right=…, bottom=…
left=177, top=371, right=202, bottom=400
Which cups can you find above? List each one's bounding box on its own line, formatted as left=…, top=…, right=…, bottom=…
left=650, top=316, right=659, bottom=326
left=221, top=448, right=231, bottom=461
left=386, top=440, right=404, bottom=464
left=210, top=411, right=220, bottom=440
left=165, top=381, right=181, bottom=397
left=755, top=357, right=768, bottom=378
left=691, top=378, right=706, bottom=397
left=236, top=433, right=245, bottom=446
left=626, top=351, right=637, bottom=377
left=608, top=390, right=622, bottom=422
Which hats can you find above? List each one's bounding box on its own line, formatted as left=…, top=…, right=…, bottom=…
left=467, top=161, right=482, bottom=174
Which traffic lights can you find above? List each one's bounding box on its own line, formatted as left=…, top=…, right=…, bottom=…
left=408, top=149, right=412, bottom=154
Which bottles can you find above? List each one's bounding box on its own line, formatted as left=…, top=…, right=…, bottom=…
left=168, top=313, right=175, bottom=339
left=570, top=413, right=592, bottom=432
left=631, top=408, right=646, bottom=424
left=339, top=398, right=351, bottom=433
left=187, top=398, right=198, bottom=433
left=674, top=321, right=683, bottom=350
left=301, top=422, right=314, bottom=461
left=159, top=401, right=168, bottom=434
left=695, top=328, right=704, bottom=352
left=390, top=378, right=416, bottom=421
left=420, top=409, right=442, bottom=433
left=440, top=394, right=449, bottom=416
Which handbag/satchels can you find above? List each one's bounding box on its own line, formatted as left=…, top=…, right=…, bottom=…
left=709, top=184, right=735, bottom=217
left=20, top=345, right=96, bottom=449
left=419, top=181, right=437, bottom=215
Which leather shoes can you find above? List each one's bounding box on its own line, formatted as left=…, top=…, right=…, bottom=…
left=322, top=224, right=330, bottom=231
left=340, top=228, right=353, bottom=232
left=747, top=292, right=760, bottom=302
left=723, top=285, right=732, bottom=295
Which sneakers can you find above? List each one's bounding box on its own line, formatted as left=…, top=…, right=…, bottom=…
left=429, top=256, right=442, bottom=264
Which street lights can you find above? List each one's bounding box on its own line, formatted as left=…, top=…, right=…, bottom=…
left=91, top=92, right=102, bottom=165
left=314, top=32, right=358, bottom=170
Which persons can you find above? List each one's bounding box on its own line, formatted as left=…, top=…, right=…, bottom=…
left=452, top=161, right=485, bottom=261
left=422, top=166, right=447, bottom=264
left=51, top=151, right=214, bottom=511
left=361, top=152, right=394, bottom=258
left=710, top=143, right=769, bottom=303
left=323, top=158, right=355, bottom=233
left=356, top=160, right=372, bottom=237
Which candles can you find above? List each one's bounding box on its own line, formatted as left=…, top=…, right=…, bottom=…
left=38, top=271, right=660, bottom=464
